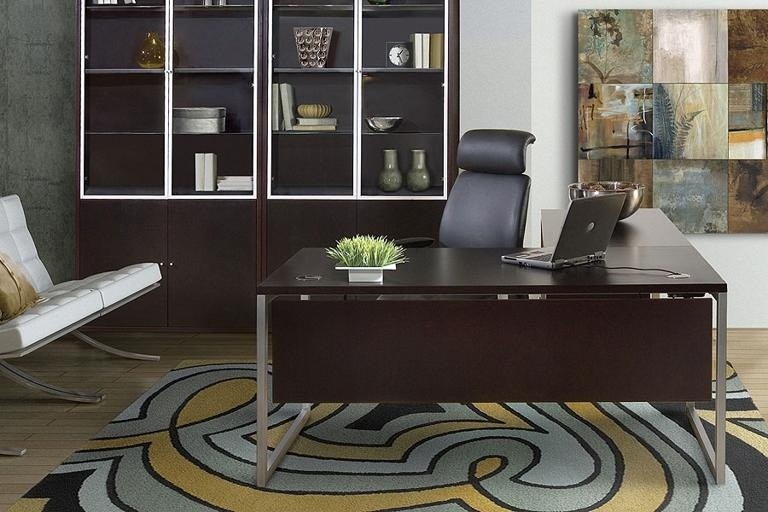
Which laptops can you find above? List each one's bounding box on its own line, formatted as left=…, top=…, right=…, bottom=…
left=500, top=193, right=628, bottom=271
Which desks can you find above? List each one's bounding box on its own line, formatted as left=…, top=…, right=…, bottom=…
left=253, top=209, right=728, bottom=489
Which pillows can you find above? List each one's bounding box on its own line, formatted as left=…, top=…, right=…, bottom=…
left=0, top=253, right=44, bottom=326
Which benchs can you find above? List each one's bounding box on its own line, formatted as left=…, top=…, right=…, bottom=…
left=2, top=195, right=162, bottom=404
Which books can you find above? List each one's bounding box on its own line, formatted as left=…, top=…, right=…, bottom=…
left=192, top=151, right=253, bottom=192
left=271, top=81, right=297, bottom=131
left=408, top=31, right=444, bottom=68
left=291, top=117, right=338, bottom=131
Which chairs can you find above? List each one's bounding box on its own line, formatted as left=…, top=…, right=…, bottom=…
left=406, top=129, right=532, bottom=249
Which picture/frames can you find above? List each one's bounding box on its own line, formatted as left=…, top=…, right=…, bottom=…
left=576, top=12, right=766, bottom=233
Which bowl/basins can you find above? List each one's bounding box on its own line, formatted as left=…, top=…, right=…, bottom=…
left=566, top=180, right=647, bottom=223
left=364, top=114, right=404, bottom=132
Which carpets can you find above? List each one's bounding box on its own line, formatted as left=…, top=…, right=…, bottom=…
left=10, top=358, right=768, bottom=512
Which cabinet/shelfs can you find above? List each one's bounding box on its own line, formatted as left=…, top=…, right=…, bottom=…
left=78, top=2, right=263, bottom=201
left=262, top=200, right=457, bottom=333
left=76, top=198, right=262, bottom=333
left=262, top=3, right=456, bottom=201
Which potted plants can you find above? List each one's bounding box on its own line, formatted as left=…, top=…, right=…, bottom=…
left=323, top=232, right=404, bottom=286
